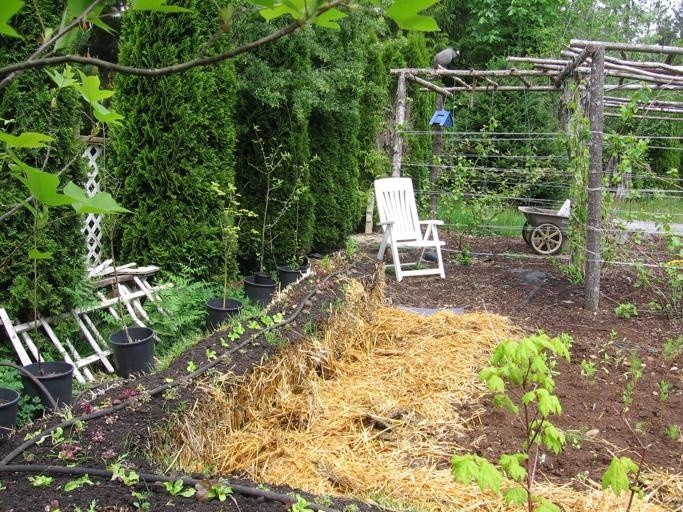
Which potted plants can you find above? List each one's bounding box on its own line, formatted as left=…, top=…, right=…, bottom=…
left=191, top=133, right=327, bottom=335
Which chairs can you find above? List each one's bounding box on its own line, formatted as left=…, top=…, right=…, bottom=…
left=372, top=175, right=448, bottom=283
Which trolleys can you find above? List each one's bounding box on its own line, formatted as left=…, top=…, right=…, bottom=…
left=515, top=204, right=571, bottom=256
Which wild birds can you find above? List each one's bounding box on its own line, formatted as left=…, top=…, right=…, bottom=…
left=429, top=47, right=460, bottom=70
